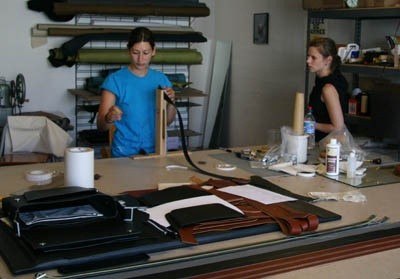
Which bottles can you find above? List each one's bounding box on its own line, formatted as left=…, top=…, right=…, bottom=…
left=325, top=139, right=340, bottom=175
left=361, top=92, right=368, bottom=114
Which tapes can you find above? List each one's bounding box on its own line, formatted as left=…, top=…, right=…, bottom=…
left=216, top=163, right=237, bottom=171
left=25, top=170, right=53, bottom=182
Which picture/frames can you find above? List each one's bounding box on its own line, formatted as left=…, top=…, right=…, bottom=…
left=253, top=12, right=270, bottom=44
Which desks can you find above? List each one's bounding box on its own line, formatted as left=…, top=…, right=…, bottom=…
left=0, top=145, right=400, bottom=279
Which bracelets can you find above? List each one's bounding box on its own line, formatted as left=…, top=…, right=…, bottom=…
left=105, top=113, right=113, bottom=124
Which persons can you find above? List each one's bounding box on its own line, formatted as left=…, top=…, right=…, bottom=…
left=305, top=36, right=349, bottom=146
left=96, top=26, right=177, bottom=157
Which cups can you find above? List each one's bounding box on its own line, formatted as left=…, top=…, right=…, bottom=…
left=287, top=135, right=308, bottom=163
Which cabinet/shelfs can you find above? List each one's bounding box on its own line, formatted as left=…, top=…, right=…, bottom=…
left=304, top=8, right=400, bottom=143
